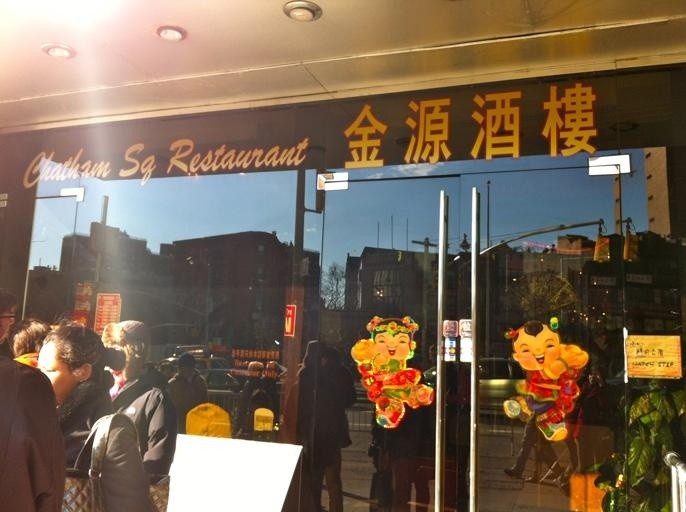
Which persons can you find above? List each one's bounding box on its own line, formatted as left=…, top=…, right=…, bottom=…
left=39, top=327, right=156, bottom=512
left=1, top=290, right=66, bottom=512
left=5, top=315, right=51, bottom=359
left=101, top=321, right=179, bottom=486
left=165, top=319, right=642, bottom=512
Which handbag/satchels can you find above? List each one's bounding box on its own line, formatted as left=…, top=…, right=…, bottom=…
left=62, top=467, right=170, bottom=512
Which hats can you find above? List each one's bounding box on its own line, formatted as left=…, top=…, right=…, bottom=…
left=103, top=320, right=149, bottom=381
